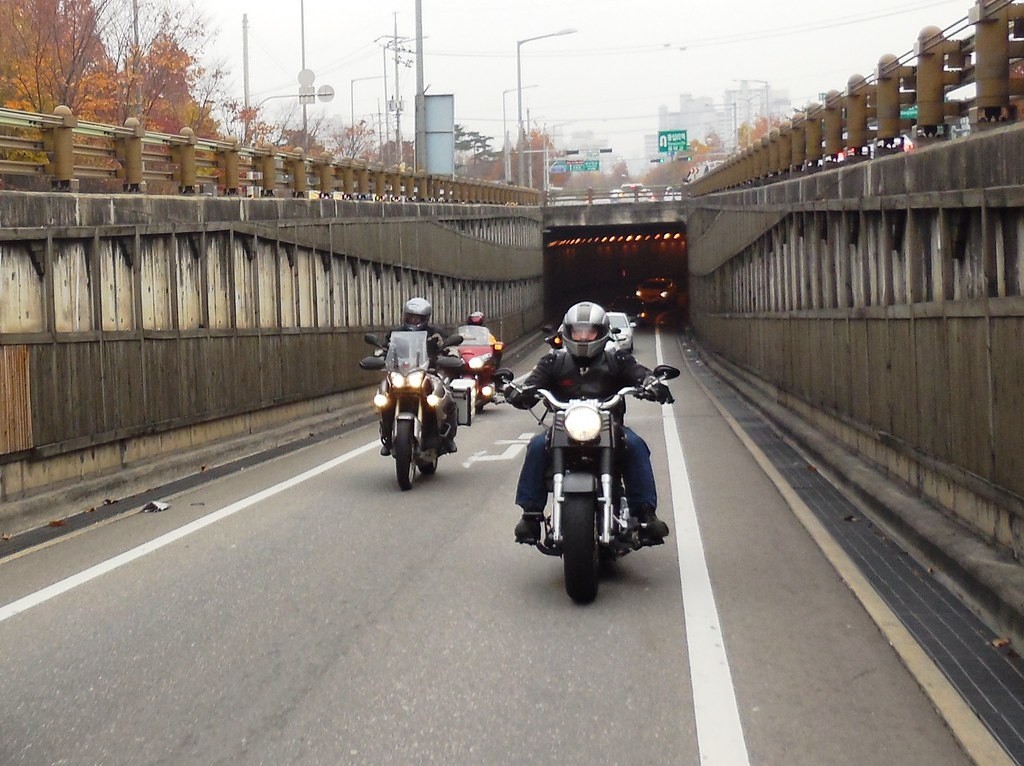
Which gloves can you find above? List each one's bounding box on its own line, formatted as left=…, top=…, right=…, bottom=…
left=506, top=384, right=531, bottom=403
left=645, top=382, right=672, bottom=405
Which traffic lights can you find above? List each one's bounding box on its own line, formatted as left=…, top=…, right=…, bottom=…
left=652, top=158, right=664, bottom=163
left=679, top=156, right=692, bottom=161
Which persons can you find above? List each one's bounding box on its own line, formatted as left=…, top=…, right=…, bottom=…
left=374, top=298, right=465, bottom=455
left=505, top=302, right=674, bottom=540
left=466, top=311, right=514, bottom=404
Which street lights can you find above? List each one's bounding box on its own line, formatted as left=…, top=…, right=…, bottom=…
left=732, top=77, right=771, bottom=122
left=516, top=27, right=581, bottom=189
left=501, top=83, right=540, bottom=184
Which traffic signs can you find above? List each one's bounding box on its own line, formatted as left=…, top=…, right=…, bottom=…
left=566, top=161, right=582, bottom=171
left=552, top=160, right=568, bottom=172
left=657, top=129, right=687, bottom=153
left=581, top=160, right=600, bottom=171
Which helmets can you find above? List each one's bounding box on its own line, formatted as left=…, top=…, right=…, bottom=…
left=402, top=297, right=431, bottom=331
left=468, top=311, right=484, bottom=326
left=562, top=301, right=610, bottom=358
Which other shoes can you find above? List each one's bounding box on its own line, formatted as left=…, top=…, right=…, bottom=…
left=443, top=437, right=457, bottom=453
left=637, top=511, right=669, bottom=537
left=515, top=515, right=540, bottom=540
left=381, top=440, right=391, bottom=456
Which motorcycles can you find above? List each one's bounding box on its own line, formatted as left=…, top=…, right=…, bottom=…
left=440, top=325, right=515, bottom=417
left=359, top=331, right=467, bottom=494
left=490, top=363, right=682, bottom=604
left=539, top=324, right=622, bottom=358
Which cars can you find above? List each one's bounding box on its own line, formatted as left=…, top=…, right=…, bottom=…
left=635, top=278, right=676, bottom=303
left=600, top=311, right=639, bottom=354
